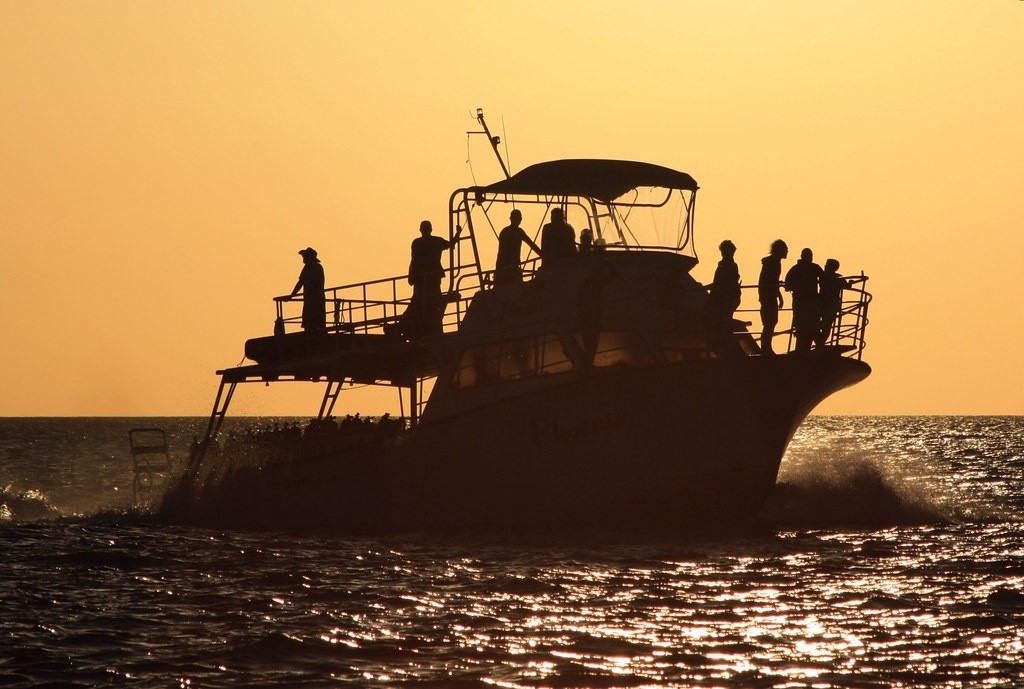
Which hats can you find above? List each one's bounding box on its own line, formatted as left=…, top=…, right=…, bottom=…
left=298, top=248, right=322, bottom=263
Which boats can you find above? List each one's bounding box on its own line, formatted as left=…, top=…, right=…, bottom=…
left=128, top=106, right=875, bottom=536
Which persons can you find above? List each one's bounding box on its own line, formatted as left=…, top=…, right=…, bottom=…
left=284, top=247, right=328, bottom=333
left=541, top=207, right=577, bottom=264
left=758, top=239, right=789, bottom=354
left=709, top=240, right=742, bottom=317
left=577, top=229, right=606, bottom=250
left=408, top=220, right=462, bottom=333
left=493, top=209, right=541, bottom=288
left=785, top=248, right=855, bottom=351
